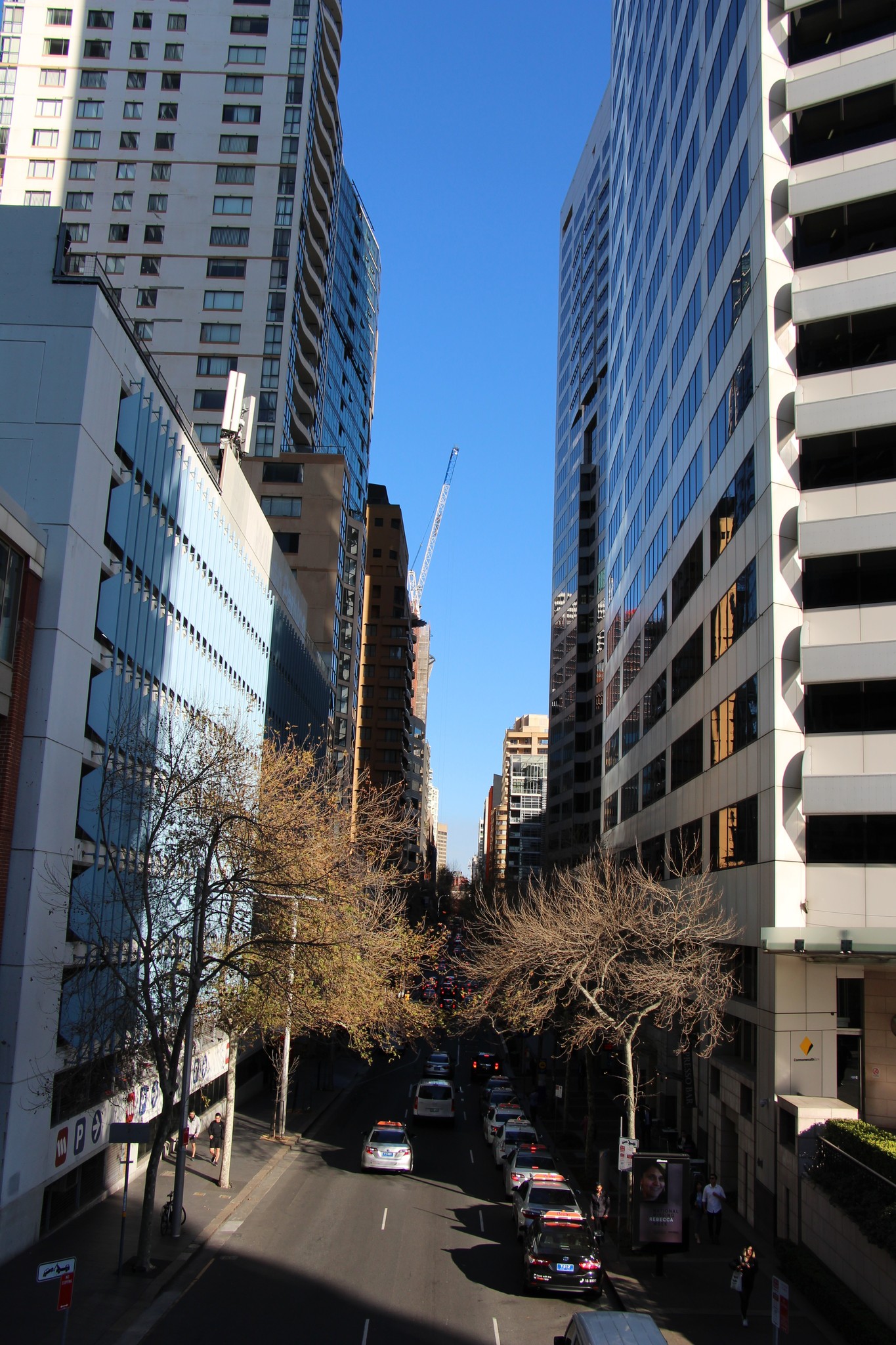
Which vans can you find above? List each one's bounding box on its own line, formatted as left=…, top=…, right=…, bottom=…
left=414, top=1078, right=459, bottom=1121
left=554, top=1310, right=668, bottom=1345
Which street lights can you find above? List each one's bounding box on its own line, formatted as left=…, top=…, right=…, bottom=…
left=166, top=869, right=324, bottom=1236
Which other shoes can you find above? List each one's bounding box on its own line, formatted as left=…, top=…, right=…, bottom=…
left=696, top=1236, right=702, bottom=1244
left=742, top=1319, right=749, bottom=1328
left=191, top=1157, right=195, bottom=1161
left=211, top=1157, right=216, bottom=1162
left=214, top=1162, right=218, bottom=1166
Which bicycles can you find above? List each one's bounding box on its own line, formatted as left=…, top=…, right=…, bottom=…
left=159, top=1190, right=186, bottom=1236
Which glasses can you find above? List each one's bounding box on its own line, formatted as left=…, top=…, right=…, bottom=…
left=709, top=1177, right=715, bottom=1180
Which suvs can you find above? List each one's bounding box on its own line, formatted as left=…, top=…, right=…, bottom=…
left=524, top=1210, right=605, bottom=1301
left=470, top=1052, right=504, bottom=1079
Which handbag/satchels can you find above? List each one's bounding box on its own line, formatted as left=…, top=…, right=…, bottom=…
left=731, top=1270, right=743, bottom=1292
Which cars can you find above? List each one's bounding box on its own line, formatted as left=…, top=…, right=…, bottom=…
left=478, top=1075, right=584, bottom=1234
left=423, top=1051, right=455, bottom=1078
left=423, top=918, right=479, bottom=1011
left=359, top=1121, right=415, bottom=1175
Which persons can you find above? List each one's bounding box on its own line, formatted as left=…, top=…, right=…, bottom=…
left=186, top=1109, right=201, bottom=1161
left=728, top=1244, right=760, bottom=1328
left=681, top=1133, right=698, bottom=1159
left=689, top=1180, right=707, bottom=1242
left=702, top=1173, right=727, bottom=1246
left=208, top=1113, right=225, bottom=1166
left=581, top=1112, right=597, bottom=1149
left=588, top=1181, right=611, bottom=1247
left=676, top=1130, right=687, bottom=1154
left=577, top=1060, right=587, bottom=1092
left=633, top=1158, right=665, bottom=1201
left=623, top=1105, right=628, bottom=1137
left=638, top=1104, right=653, bottom=1151
left=528, top=1086, right=538, bottom=1121
